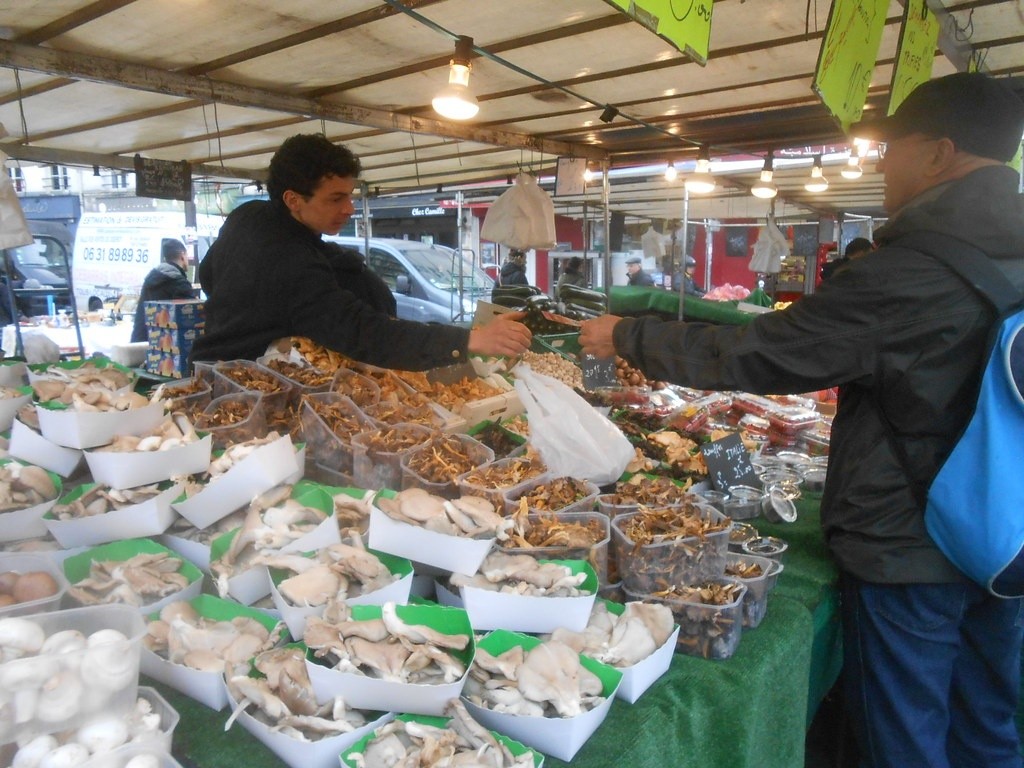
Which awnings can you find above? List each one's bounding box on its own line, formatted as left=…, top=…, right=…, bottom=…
left=350, top=192, right=457, bottom=219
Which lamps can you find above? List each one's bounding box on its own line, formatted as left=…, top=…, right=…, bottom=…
left=431, top=35, right=480, bottom=119
left=840, top=144, right=863, bottom=180
left=804, top=153, right=829, bottom=192
left=684, top=140, right=716, bottom=194
left=751, top=149, right=779, bottom=199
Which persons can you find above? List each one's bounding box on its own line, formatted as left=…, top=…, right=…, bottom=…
left=671, top=255, right=706, bottom=297
left=578, top=70, right=1024, bottom=768
left=492, top=248, right=529, bottom=286
left=188, top=132, right=533, bottom=375
left=820, top=238, right=874, bottom=281
left=0, top=282, right=29, bottom=348
left=554, top=256, right=586, bottom=300
left=130, top=238, right=196, bottom=343
left=625, top=258, right=654, bottom=286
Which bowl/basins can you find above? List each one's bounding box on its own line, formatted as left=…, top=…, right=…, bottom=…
left=0, top=355, right=785, bottom=768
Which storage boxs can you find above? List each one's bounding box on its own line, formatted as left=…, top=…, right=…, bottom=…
left=0, top=299, right=836, bottom=768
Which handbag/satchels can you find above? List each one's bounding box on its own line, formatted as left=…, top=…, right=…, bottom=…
left=512, top=363, right=633, bottom=488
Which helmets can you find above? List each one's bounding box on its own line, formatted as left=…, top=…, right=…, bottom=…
left=680, top=255, right=696, bottom=267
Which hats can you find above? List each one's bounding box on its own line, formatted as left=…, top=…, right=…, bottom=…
left=626, top=257, right=642, bottom=265
left=848, top=71, right=1024, bottom=162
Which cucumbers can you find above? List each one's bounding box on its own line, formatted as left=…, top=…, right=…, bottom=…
left=492, top=283, right=607, bottom=335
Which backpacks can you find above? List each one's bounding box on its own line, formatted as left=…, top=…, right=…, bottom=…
left=883, top=230, right=1024, bottom=601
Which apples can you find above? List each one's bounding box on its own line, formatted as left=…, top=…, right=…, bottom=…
left=818, top=386, right=839, bottom=403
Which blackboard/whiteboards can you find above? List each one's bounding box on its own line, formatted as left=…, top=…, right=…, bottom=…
left=581, top=352, right=617, bottom=389
left=793, top=225, right=819, bottom=255
left=727, top=227, right=748, bottom=257
left=134, top=155, right=191, bottom=202
left=841, top=221, right=870, bottom=256
left=701, top=433, right=762, bottom=500
left=555, top=157, right=586, bottom=197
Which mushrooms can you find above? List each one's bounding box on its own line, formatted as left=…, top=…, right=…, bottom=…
left=0, top=337, right=773, bottom=768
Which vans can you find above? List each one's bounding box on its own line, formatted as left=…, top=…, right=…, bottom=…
left=0, top=233, right=71, bottom=317
left=324, top=236, right=494, bottom=327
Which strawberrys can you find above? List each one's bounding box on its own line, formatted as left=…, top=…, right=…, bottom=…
left=613, top=389, right=829, bottom=453
